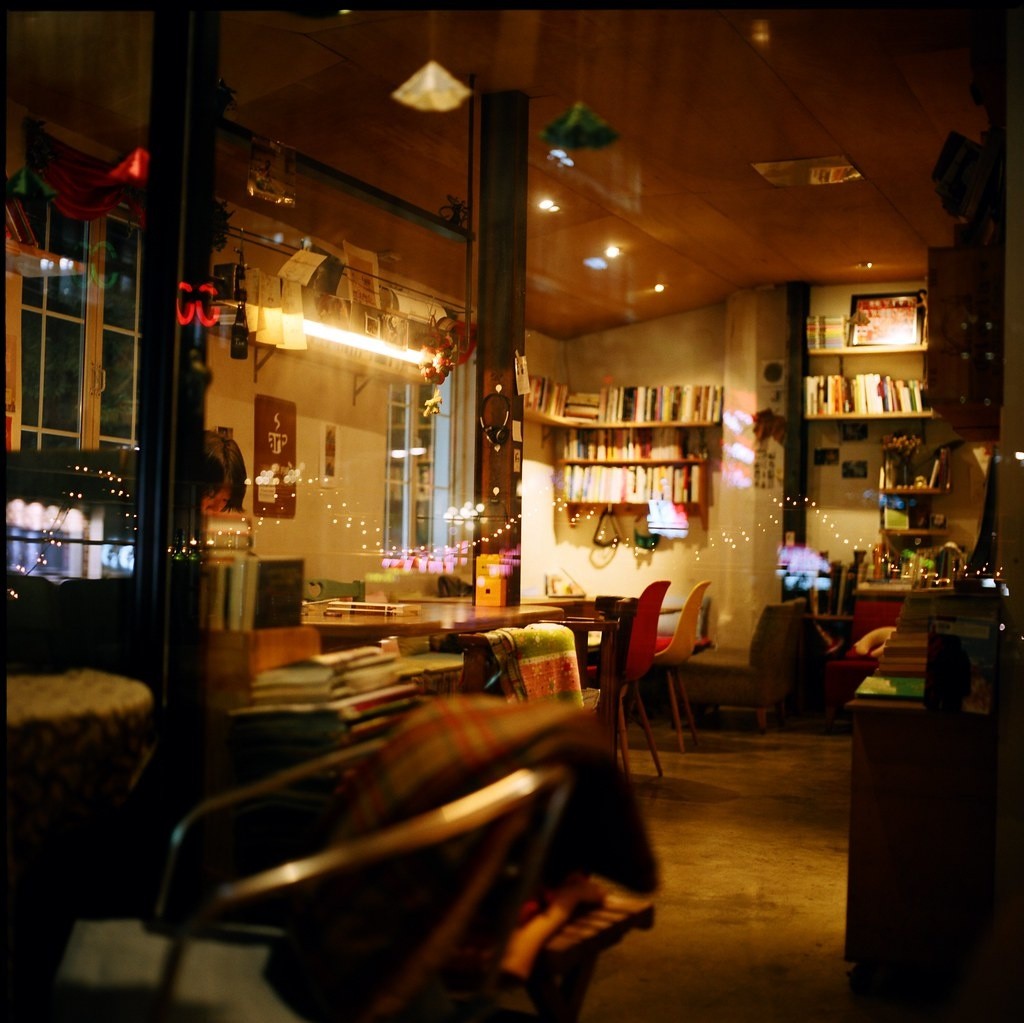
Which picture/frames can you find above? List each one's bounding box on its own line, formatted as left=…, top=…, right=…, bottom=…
left=848, top=289, right=923, bottom=348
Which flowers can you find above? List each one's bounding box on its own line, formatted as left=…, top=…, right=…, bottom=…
left=879, top=430, right=923, bottom=465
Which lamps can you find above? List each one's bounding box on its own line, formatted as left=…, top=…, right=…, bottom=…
left=107, top=11, right=150, bottom=190
left=6, top=11, right=56, bottom=198
left=391, top=10, right=472, bottom=113
left=445, top=487, right=502, bottom=532
left=538, top=10, right=623, bottom=153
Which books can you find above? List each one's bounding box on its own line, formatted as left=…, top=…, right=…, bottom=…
left=526, top=381, right=722, bottom=505
left=854, top=587, right=956, bottom=698
left=806, top=317, right=934, bottom=417
left=230, top=646, right=417, bottom=794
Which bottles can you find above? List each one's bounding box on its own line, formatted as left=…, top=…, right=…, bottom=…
left=6, top=498, right=104, bottom=580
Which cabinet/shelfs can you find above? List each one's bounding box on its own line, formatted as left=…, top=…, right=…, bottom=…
left=523, top=405, right=715, bottom=531
left=876, top=488, right=951, bottom=537
left=803, top=345, right=932, bottom=420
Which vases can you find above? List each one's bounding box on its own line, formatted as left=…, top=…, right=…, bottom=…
left=897, top=458, right=915, bottom=485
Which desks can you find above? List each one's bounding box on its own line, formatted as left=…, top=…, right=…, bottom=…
left=300, top=604, right=563, bottom=640
left=5, top=669, right=157, bottom=904
left=843, top=699, right=998, bottom=1004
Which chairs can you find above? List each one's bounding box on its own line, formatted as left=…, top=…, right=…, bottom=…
left=51, top=693, right=662, bottom=1023
left=823, top=600, right=905, bottom=733
left=455, top=580, right=805, bottom=786
